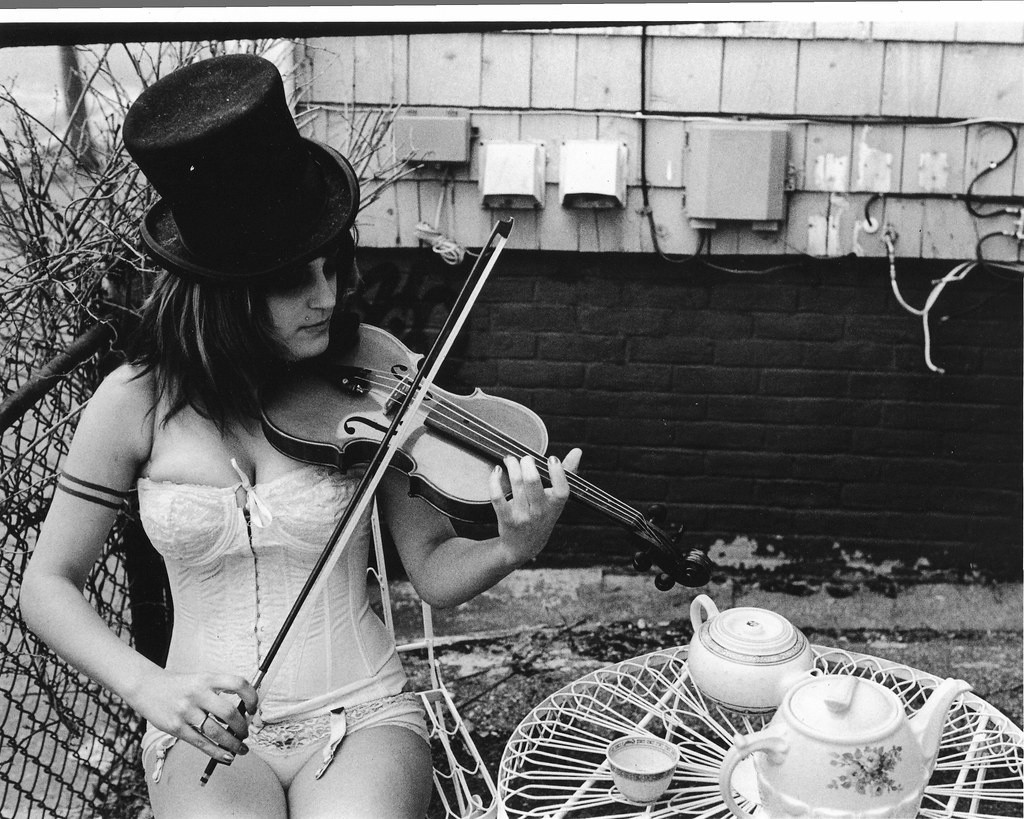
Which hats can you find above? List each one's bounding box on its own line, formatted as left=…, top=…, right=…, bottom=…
left=122, top=54, right=361, bottom=287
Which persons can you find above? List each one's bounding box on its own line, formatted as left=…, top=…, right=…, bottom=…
left=20, top=54, right=583, bottom=819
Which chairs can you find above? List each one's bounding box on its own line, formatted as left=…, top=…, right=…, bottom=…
left=366, top=489, right=511, bottom=819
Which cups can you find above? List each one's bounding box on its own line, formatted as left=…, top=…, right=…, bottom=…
left=606, top=735, right=680, bottom=803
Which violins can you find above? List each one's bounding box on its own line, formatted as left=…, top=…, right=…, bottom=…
left=258, top=316, right=717, bottom=593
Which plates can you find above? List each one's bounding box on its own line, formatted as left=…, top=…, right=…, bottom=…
left=731, top=751, right=761, bottom=805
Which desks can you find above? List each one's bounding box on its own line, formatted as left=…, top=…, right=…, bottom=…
left=497, top=645, right=1023, bottom=818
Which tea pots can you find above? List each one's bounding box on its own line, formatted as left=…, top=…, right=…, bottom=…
left=718, top=675, right=973, bottom=819
left=687, top=593, right=826, bottom=712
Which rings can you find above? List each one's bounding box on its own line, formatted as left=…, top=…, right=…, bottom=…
left=193, top=714, right=211, bottom=733
